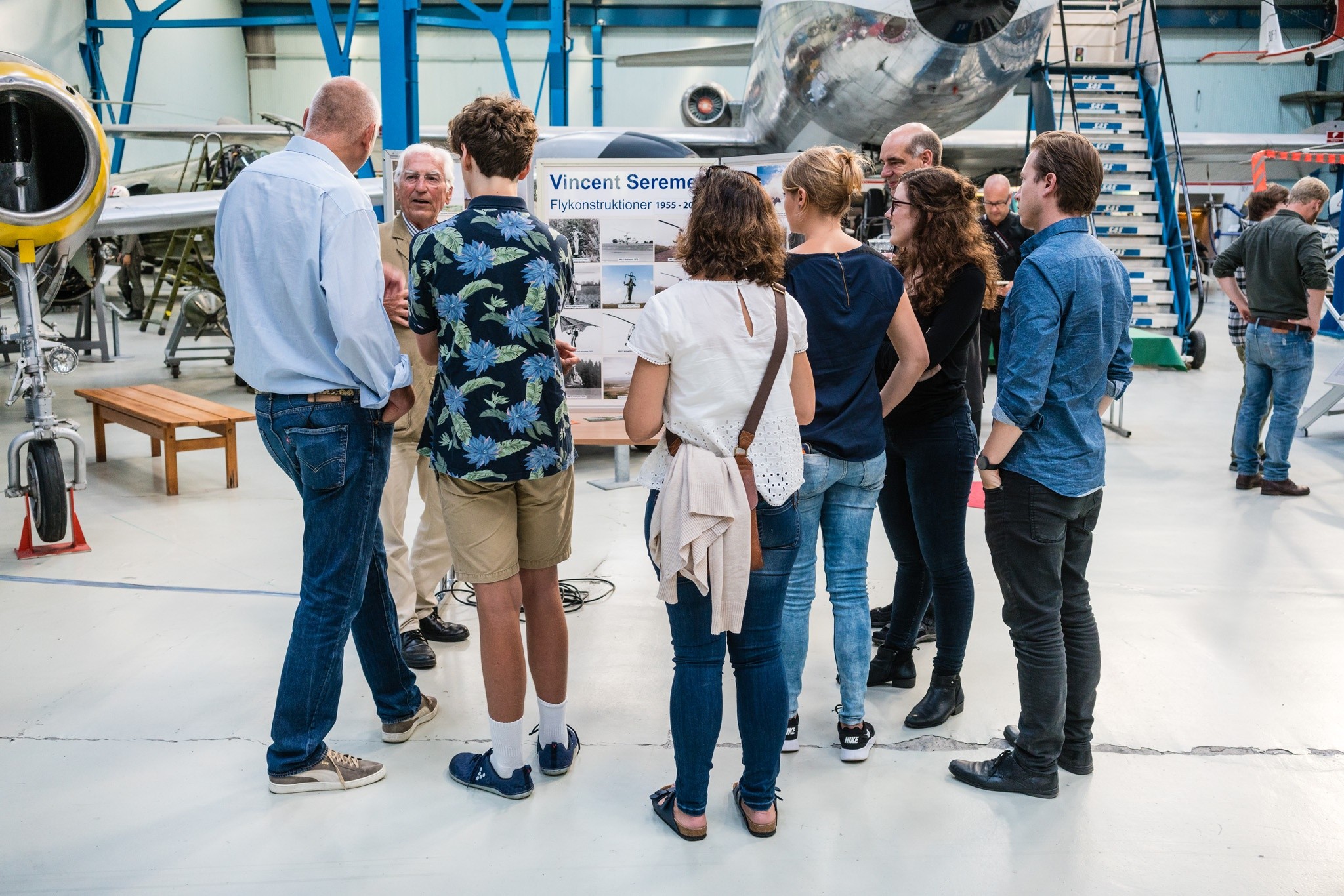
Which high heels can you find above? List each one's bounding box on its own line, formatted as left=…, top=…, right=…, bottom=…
left=835, top=643, right=917, bottom=688
left=904, top=670, right=964, bottom=729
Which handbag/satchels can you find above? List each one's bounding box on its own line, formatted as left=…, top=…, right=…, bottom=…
left=734, top=454, right=763, bottom=570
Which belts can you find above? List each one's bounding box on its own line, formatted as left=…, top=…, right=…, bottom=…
left=1249, top=318, right=1312, bottom=331
left=254, top=388, right=360, bottom=396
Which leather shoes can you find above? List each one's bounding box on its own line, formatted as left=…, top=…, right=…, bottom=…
left=1261, top=478, right=1310, bottom=495
left=1236, top=473, right=1264, bottom=489
left=948, top=750, right=1059, bottom=799
left=399, top=629, right=436, bottom=667
left=419, top=610, right=469, bottom=642
left=1003, top=725, right=1093, bottom=775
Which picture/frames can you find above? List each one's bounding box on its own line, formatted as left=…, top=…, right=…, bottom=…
left=719, top=149, right=804, bottom=248
left=534, top=155, right=720, bottom=413
left=382, top=147, right=472, bottom=226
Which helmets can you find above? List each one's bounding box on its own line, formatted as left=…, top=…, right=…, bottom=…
left=109, top=186, right=130, bottom=200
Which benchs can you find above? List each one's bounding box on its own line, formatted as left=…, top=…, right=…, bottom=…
left=564, top=411, right=670, bottom=492
left=75, top=381, right=257, bottom=494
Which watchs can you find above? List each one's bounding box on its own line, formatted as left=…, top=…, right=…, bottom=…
left=977, top=452, right=1003, bottom=471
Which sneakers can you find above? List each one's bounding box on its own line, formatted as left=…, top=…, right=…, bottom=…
left=267, top=747, right=385, bottom=794
left=832, top=720, right=876, bottom=761
left=449, top=748, right=534, bottom=799
left=537, top=724, right=581, bottom=775
left=381, top=693, right=438, bottom=742
left=780, top=713, right=800, bottom=751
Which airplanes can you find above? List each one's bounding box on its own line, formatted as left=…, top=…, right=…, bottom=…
left=419, top=1, right=1059, bottom=348
left=0, top=50, right=384, bottom=543
left=109, top=111, right=383, bottom=341
left=1195, top=0, right=1344, bottom=65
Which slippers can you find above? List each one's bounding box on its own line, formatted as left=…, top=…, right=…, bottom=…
left=649, top=784, right=707, bottom=841
left=733, top=782, right=783, bottom=838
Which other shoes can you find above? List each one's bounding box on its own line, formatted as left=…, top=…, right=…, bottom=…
left=870, top=604, right=892, bottom=627
left=1230, top=461, right=1264, bottom=474
left=872, top=605, right=935, bottom=645
left=119, top=309, right=142, bottom=320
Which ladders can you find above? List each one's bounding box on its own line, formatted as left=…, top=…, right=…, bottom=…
left=137, top=130, right=225, bottom=336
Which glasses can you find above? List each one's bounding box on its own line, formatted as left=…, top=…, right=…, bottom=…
left=891, top=198, right=915, bottom=215
left=1271, top=198, right=1289, bottom=207
left=706, top=165, right=761, bottom=184
left=399, top=171, right=450, bottom=188
left=983, top=194, right=1010, bottom=207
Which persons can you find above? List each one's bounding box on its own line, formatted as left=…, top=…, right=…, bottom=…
left=1224, top=181, right=1293, bottom=471
left=943, top=129, right=1140, bottom=806
left=374, top=138, right=472, bottom=675
left=863, top=120, right=943, bottom=648
left=214, top=74, right=440, bottom=796
left=977, top=170, right=1034, bottom=435
left=413, top=88, right=582, bottom=799
left=1215, top=169, right=1334, bottom=497
left=108, top=183, right=146, bottom=322
left=623, top=165, right=824, bottom=842
left=772, top=142, right=936, bottom=764
left=834, top=163, right=1004, bottom=734
